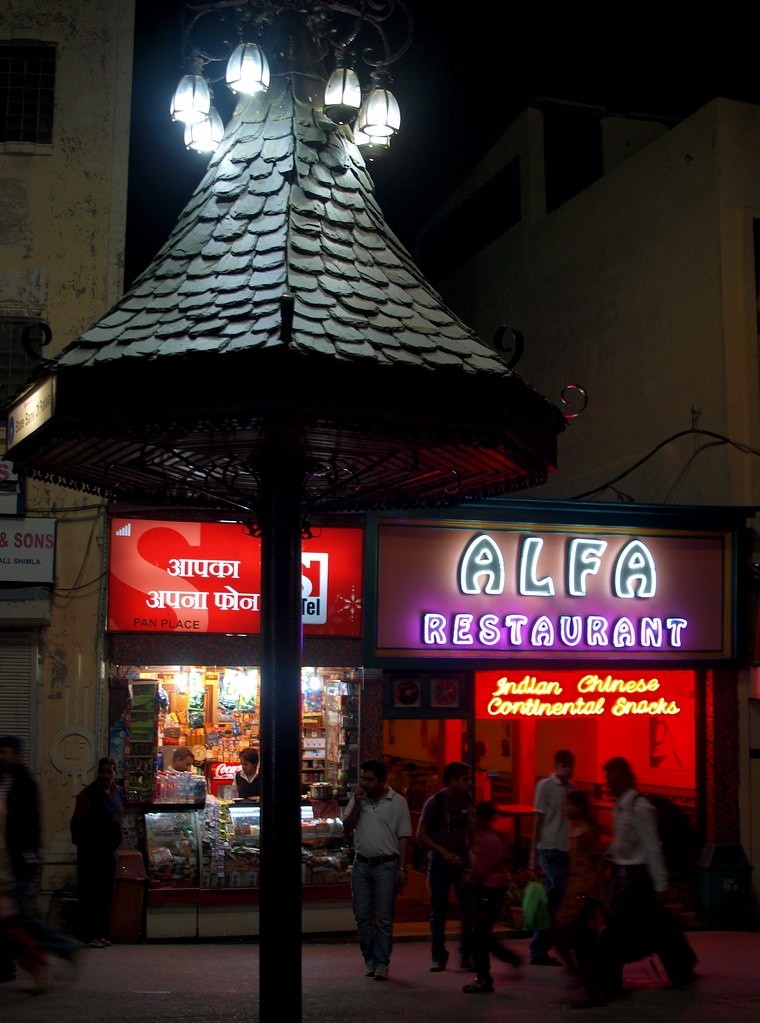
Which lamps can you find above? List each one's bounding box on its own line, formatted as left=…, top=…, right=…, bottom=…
left=168, top=1, right=402, bottom=159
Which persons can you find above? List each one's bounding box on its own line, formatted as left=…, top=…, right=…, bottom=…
left=164, top=747, right=195, bottom=773
left=231, top=748, right=261, bottom=803
left=415, top=749, right=700, bottom=1008
left=69, top=756, right=124, bottom=948
left=0, top=737, right=91, bottom=993
left=342, top=760, right=412, bottom=978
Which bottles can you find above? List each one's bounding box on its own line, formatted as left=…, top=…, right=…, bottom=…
left=157, top=750, right=164, bottom=769
left=179, top=732, right=185, bottom=746
left=150, top=770, right=207, bottom=803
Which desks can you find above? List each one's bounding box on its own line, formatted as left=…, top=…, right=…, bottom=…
left=492, top=804, right=535, bottom=870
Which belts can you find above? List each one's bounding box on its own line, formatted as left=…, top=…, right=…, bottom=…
left=356, top=853, right=395, bottom=864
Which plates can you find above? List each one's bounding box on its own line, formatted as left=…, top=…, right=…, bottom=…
left=192, top=746, right=207, bottom=761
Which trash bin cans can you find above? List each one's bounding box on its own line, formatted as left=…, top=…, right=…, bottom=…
left=697, top=841, right=755, bottom=931
left=108, top=850, right=146, bottom=944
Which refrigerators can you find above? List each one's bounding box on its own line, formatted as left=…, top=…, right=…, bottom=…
left=206, top=762, right=240, bottom=798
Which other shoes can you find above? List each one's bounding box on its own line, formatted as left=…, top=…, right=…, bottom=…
left=530, top=956, right=556, bottom=964
left=97, top=938, right=112, bottom=946
left=73, top=936, right=105, bottom=947
left=430, top=960, right=446, bottom=970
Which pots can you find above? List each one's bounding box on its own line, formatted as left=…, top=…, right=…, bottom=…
left=309, top=781, right=334, bottom=800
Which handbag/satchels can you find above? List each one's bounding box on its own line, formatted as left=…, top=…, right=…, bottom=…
left=522, top=872, right=550, bottom=930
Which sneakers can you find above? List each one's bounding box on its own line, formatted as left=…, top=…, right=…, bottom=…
left=365, top=961, right=373, bottom=974
left=373, top=963, right=387, bottom=979
left=462, top=975, right=492, bottom=992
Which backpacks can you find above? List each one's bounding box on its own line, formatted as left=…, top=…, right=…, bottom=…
left=631, top=792, right=693, bottom=877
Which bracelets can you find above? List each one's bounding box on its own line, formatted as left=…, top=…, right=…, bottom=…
left=399, top=866, right=409, bottom=872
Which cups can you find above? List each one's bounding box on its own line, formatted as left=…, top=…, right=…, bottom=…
left=302, top=772, right=325, bottom=783
